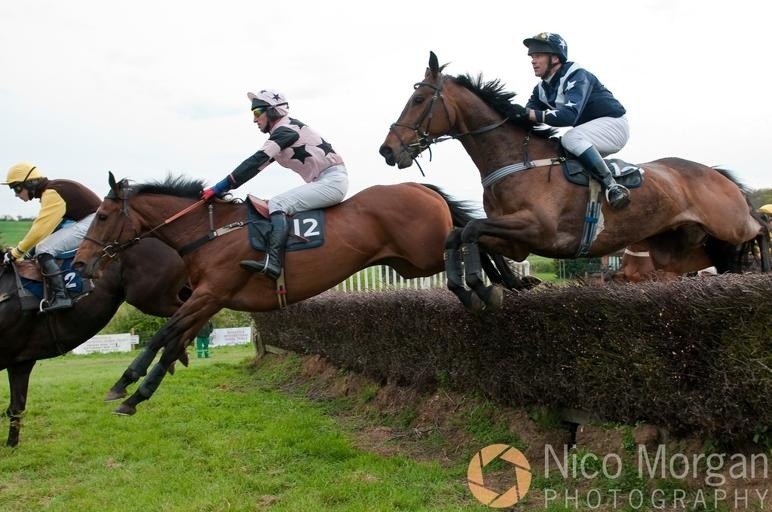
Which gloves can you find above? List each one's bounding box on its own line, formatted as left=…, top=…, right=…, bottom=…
left=3, top=252, right=15, bottom=266
left=197, top=173, right=236, bottom=204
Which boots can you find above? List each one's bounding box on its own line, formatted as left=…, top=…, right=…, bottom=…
left=239, top=209, right=290, bottom=281
left=578, top=145, right=631, bottom=209
left=37, top=253, right=73, bottom=312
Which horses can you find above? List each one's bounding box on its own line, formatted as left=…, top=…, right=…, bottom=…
left=71, top=171, right=542, bottom=416
left=378, top=51, right=771, bottom=315
left=0, top=236, right=195, bottom=447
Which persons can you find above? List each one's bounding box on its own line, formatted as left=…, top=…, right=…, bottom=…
left=0, top=161, right=104, bottom=315
left=201, top=88, right=349, bottom=281
left=197, top=320, right=213, bottom=357
left=510, top=33, right=631, bottom=211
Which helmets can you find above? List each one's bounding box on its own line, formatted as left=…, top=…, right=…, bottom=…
left=1, top=162, right=42, bottom=185
left=522, top=31, right=568, bottom=61
left=248, top=88, right=290, bottom=117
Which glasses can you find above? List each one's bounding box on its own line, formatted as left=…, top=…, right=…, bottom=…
left=13, top=186, right=25, bottom=194
left=251, top=106, right=269, bottom=116
